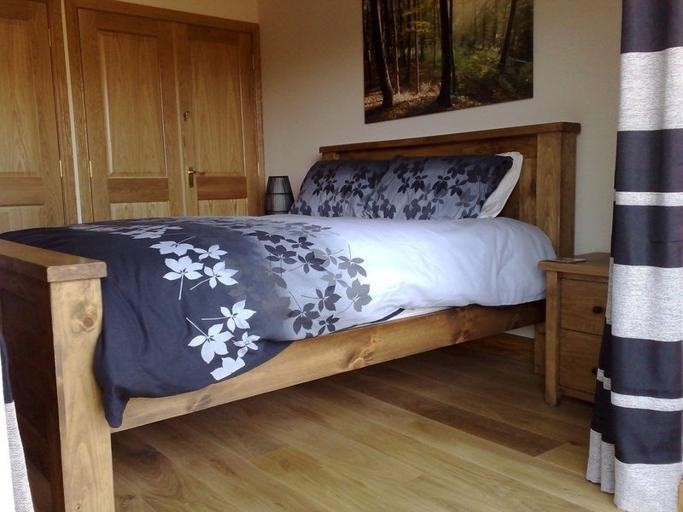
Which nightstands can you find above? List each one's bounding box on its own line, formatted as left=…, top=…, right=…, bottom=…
left=538, top=252, right=611, bottom=406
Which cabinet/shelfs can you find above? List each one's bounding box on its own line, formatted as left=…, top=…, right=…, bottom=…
left=64, top=1, right=263, bottom=222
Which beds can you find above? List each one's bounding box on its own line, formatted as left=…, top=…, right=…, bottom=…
left=1, top=122, right=580, bottom=512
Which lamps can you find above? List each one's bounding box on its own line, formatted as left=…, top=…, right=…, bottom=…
left=263, top=176, right=294, bottom=215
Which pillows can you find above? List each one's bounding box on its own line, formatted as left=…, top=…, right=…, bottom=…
left=289, top=152, right=523, bottom=219
